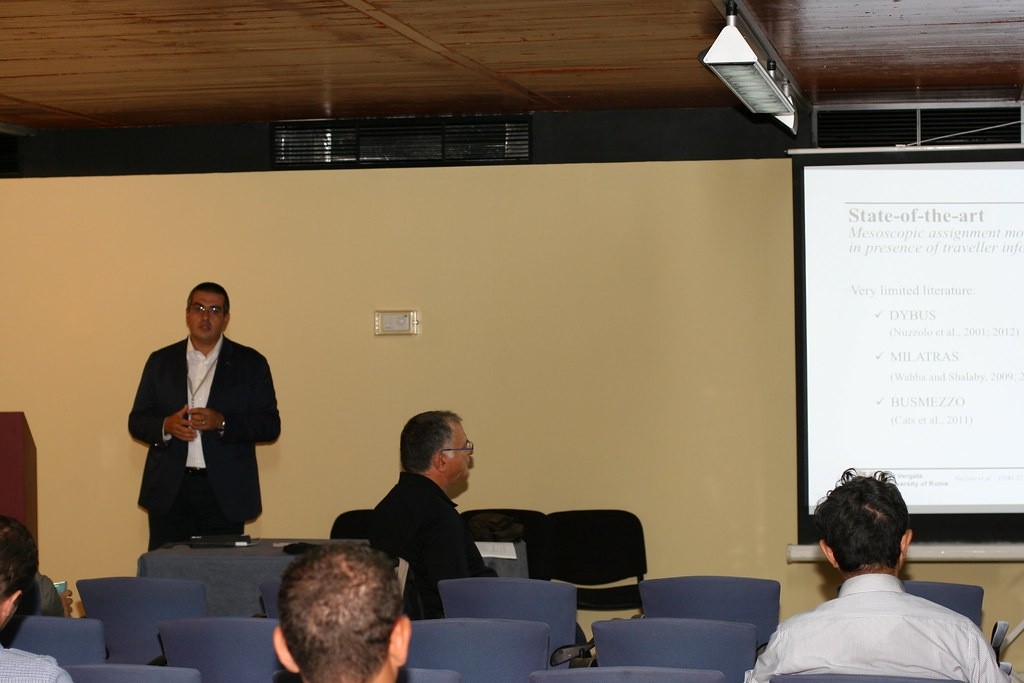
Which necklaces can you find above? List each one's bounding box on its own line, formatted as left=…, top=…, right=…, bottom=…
left=186, top=356, right=219, bottom=409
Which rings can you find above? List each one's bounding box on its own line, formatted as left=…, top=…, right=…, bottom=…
left=203, top=420, right=205, bottom=425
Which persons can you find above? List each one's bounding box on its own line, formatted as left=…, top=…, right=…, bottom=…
left=1, top=515, right=74, bottom=683
left=127, top=281, right=282, bottom=553
left=272, top=539, right=412, bottom=683
left=361, top=410, right=498, bottom=622
left=743, top=467, right=1018, bottom=683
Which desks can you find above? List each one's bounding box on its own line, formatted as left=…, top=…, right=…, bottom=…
left=144, top=532, right=368, bottom=614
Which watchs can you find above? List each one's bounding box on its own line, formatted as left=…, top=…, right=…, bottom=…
left=221, top=417, right=225, bottom=432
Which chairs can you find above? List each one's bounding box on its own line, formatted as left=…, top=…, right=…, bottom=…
left=527, top=506, right=651, bottom=608
left=437, top=577, right=582, bottom=669
left=1, top=612, right=109, bottom=667
left=460, top=505, right=546, bottom=547
left=636, top=573, right=783, bottom=641
left=407, top=619, right=551, bottom=683
left=591, top=616, right=759, bottom=683
left=157, top=617, right=294, bottom=683
left=64, top=659, right=206, bottom=683
left=898, top=580, right=984, bottom=628
left=71, top=577, right=213, bottom=670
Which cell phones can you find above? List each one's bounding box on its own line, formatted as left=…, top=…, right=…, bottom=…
left=52, top=580, right=67, bottom=593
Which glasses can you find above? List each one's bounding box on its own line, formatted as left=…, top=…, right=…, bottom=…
left=189, top=304, right=224, bottom=315
left=440, top=442, right=475, bottom=455
